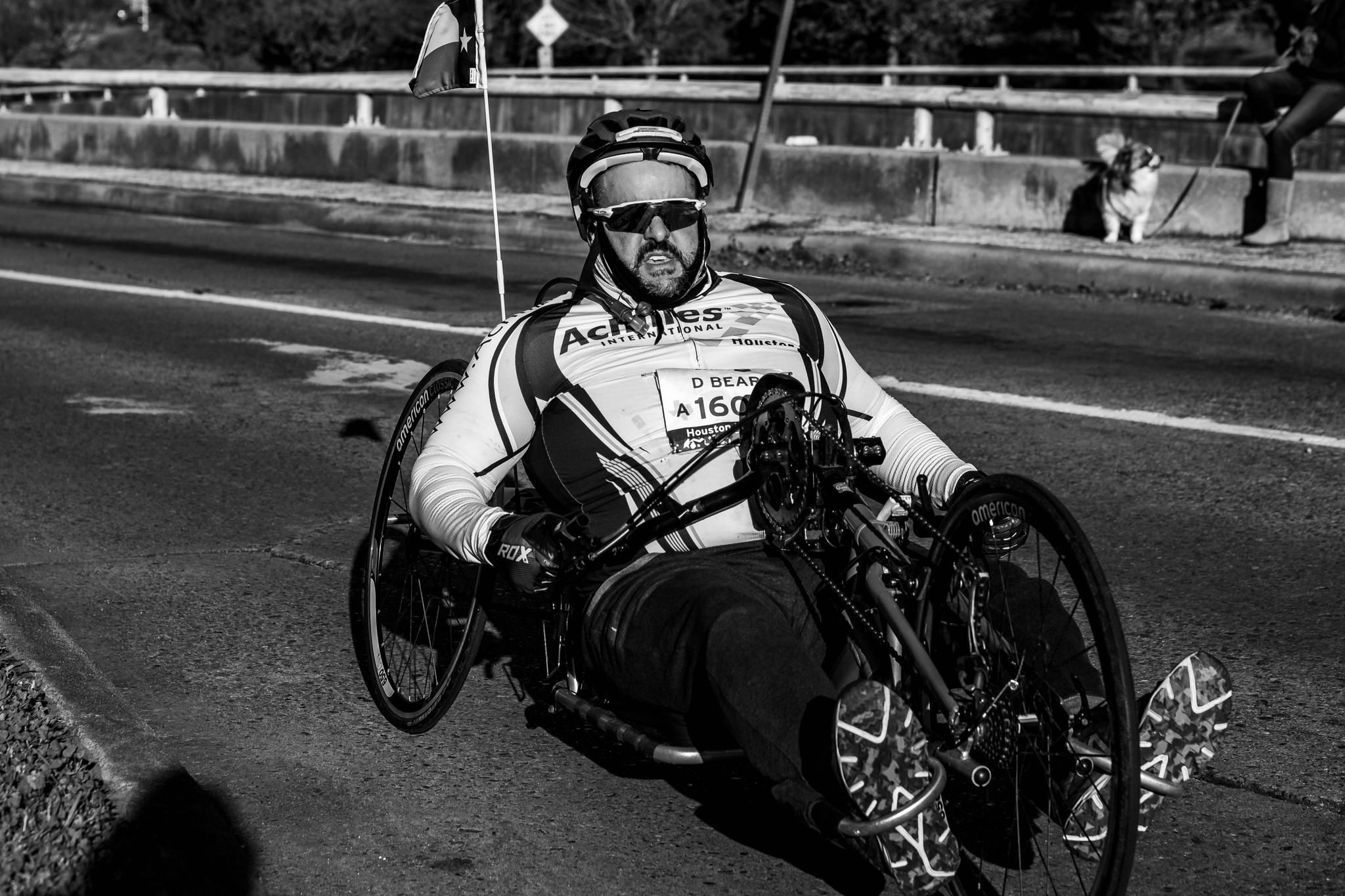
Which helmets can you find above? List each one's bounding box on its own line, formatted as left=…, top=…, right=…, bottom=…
left=565, top=106, right=715, bottom=241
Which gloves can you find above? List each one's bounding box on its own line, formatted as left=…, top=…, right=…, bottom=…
left=949, top=469, right=1033, bottom=557
left=489, top=511, right=582, bottom=599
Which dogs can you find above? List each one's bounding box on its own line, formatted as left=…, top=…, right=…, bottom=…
left=1096, top=129, right=1167, bottom=245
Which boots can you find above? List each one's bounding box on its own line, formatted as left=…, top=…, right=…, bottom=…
left=1243, top=177, right=1295, bottom=245
left=1257, top=115, right=1297, bottom=186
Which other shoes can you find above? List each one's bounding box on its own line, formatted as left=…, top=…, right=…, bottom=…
left=1061, top=651, right=1235, bottom=863
left=835, top=677, right=963, bottom=896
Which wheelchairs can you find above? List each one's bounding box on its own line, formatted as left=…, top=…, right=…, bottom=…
left=349, top=278, right=1197, bottom=896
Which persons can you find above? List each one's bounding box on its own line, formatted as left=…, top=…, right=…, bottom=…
left=408, top=106, right=1235, bottom=896
left=1240, top=0, right=1345, bottom=245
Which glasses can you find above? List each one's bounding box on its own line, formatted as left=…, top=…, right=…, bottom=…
left=586, top=196, right=706, bottom=233
